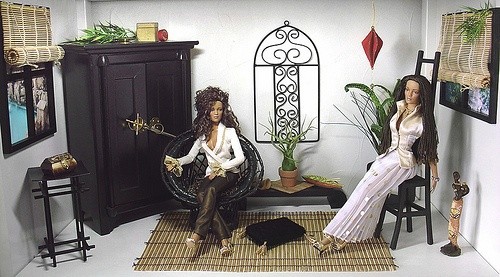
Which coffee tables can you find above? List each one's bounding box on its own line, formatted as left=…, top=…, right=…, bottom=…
left=245, top=180, right=348, bottom=210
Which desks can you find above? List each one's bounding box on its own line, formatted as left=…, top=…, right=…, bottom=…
left=26, top=163, right=95, bottom=266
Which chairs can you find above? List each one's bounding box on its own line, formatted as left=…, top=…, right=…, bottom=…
left=161, top=126, right=264, bottom=232
left=371, top=50, right=440, bottom=249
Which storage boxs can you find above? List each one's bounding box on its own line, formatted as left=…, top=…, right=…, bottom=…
left=136, top=22, right=160, bottom=42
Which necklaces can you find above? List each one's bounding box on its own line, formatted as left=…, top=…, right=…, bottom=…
left=405, top=108, right=413, bottom=115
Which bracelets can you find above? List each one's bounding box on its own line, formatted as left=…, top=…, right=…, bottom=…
left=432, top=176, right=439, bottom=182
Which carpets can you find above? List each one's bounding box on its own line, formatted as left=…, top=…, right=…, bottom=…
left=130, top=209, right=402, bottom=273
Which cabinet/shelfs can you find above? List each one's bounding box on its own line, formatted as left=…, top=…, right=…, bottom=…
left=60, top=37, right=199, bottom=237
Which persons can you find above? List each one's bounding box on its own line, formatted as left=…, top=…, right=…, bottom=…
left=313, top=75, right=439, bottom=251
left=448, top=171, right=469, bottom=245
left=164, top=87, right=246, bottom=254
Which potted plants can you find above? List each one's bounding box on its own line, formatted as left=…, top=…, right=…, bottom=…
left=258, top=110, right=318, bottom=188
